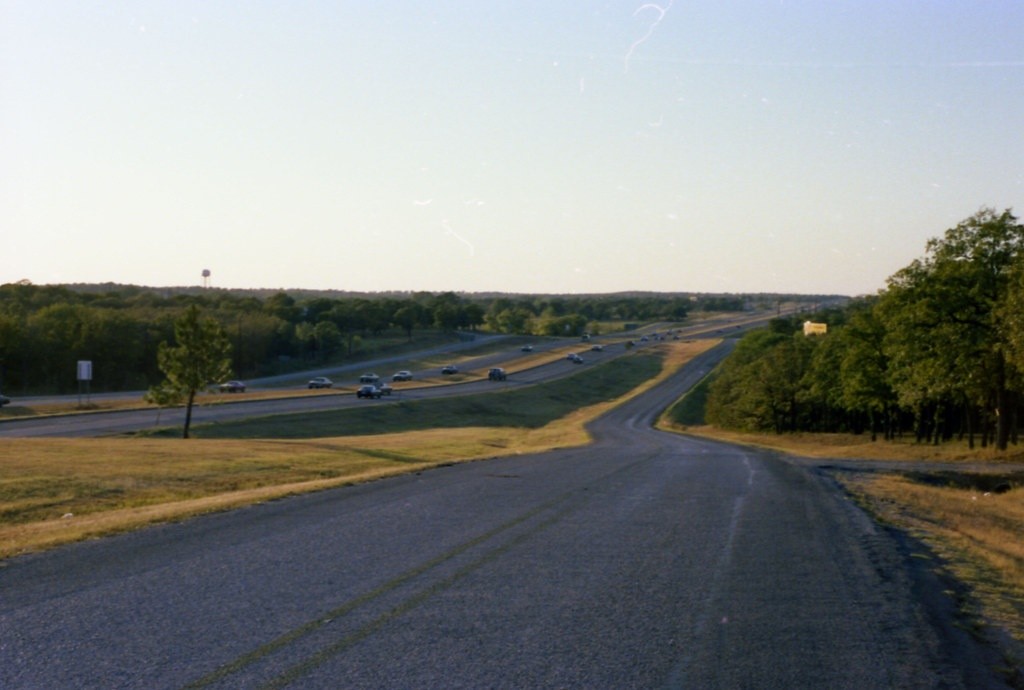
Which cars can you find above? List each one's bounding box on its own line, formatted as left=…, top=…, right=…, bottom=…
left=566, top=354, right=584, bottom=363
left=308, top=376, right=333, bottom=389
left=442, top=365, right=458, bottom=374
left=219, top=380, right=246, bottom=393
left=359, top=372, right=380, bottom=382
left=392, top=370, right=413, bottom=382
left=356, top=382, right=392, bottom=399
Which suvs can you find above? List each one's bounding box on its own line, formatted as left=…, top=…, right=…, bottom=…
left=488, top=368, right=507, bottom=381
